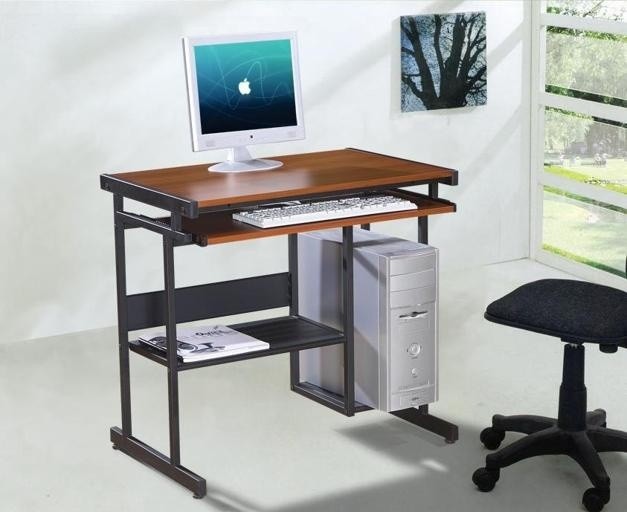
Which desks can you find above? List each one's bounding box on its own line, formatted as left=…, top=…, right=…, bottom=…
left=102, top=147, right=458, bottom=500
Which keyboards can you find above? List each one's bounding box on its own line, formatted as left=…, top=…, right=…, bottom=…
left=232, top=194, right=418, bottom=229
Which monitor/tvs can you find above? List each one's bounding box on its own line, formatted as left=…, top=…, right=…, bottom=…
left=182, top=30, right=305, bottom=172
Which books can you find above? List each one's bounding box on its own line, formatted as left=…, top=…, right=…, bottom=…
left=137, top=324, right=271, bottom=364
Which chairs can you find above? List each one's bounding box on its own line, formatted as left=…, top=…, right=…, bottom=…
left=476, top=279, right=627, bottom=512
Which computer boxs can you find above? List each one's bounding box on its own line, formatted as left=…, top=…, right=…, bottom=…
left=298, top=227, right=439, bottom=412
